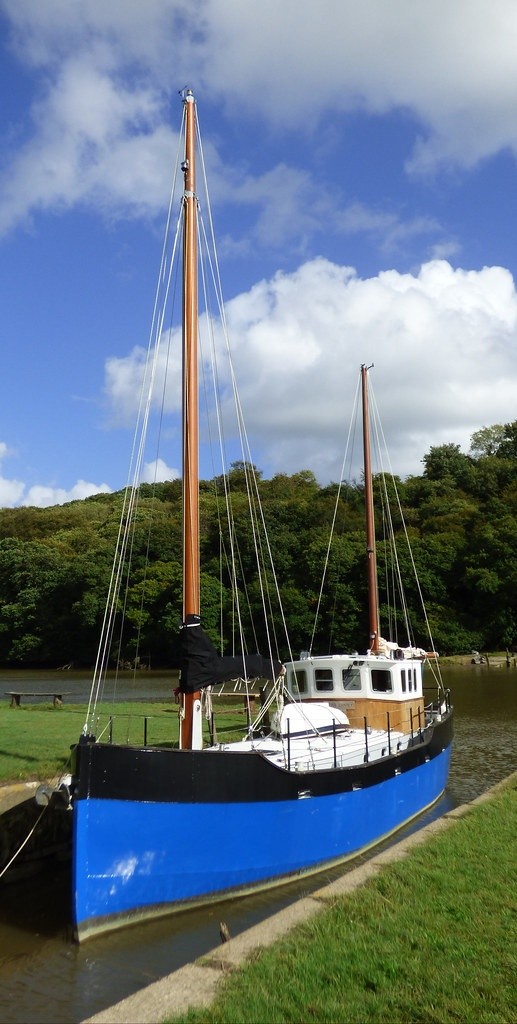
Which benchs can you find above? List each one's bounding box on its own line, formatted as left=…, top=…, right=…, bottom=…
left=4, top=691, right=72, bottom=707
left=202, top=692, right=260, bottom=712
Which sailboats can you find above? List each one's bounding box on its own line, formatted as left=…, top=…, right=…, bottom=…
left=0, top=85, right=455, bottom=946
